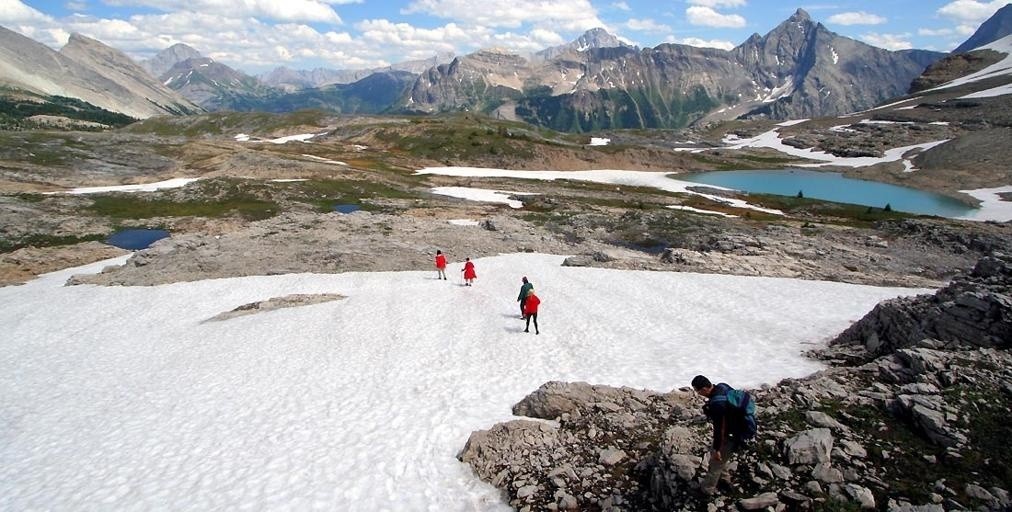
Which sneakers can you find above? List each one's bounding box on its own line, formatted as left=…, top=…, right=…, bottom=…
left=688, top=487, right=712, bottom=501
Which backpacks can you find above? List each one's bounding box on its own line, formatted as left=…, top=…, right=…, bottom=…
left=710, top=384, right=757, bottom=439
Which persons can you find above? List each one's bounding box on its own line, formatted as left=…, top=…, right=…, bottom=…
left=517, top=276, right=534, bottom=320
left=461, top=258, right=477, bottom=286
left=687, top=375, right=758, bottom=505
left=522, top=288, right=541, bottom=335
left=434, top=249, right=447, bottom=280
